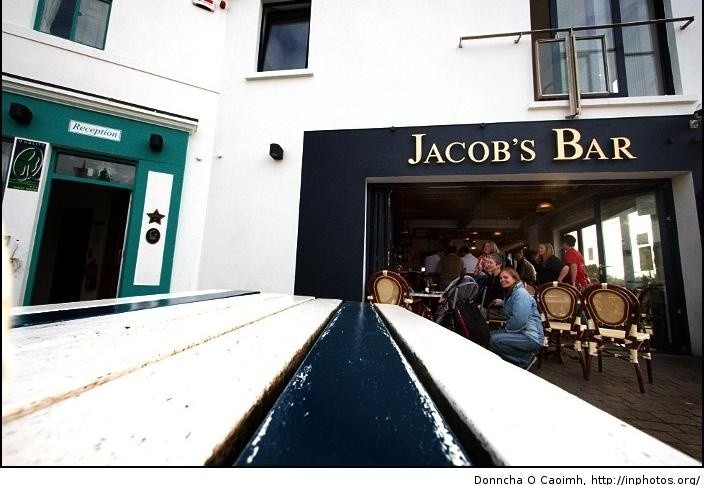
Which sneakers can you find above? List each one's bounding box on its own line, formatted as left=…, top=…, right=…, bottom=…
left=524, top=353, right=537, bottom=370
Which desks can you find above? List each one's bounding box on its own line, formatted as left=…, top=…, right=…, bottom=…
left=409, top=291, right=443, bottom=314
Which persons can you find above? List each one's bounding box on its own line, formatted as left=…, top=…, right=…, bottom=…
left=418, top=226, right=592, bottom=371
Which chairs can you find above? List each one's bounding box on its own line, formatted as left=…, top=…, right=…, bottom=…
left=480, top=299, right=509, bottom=330
left=533, top=279, right=659, bottom=394
left=368, top=269, right=425, bottom=316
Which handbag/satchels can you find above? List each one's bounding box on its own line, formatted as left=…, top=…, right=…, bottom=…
left=437, top=298, right=493, bottom=345
